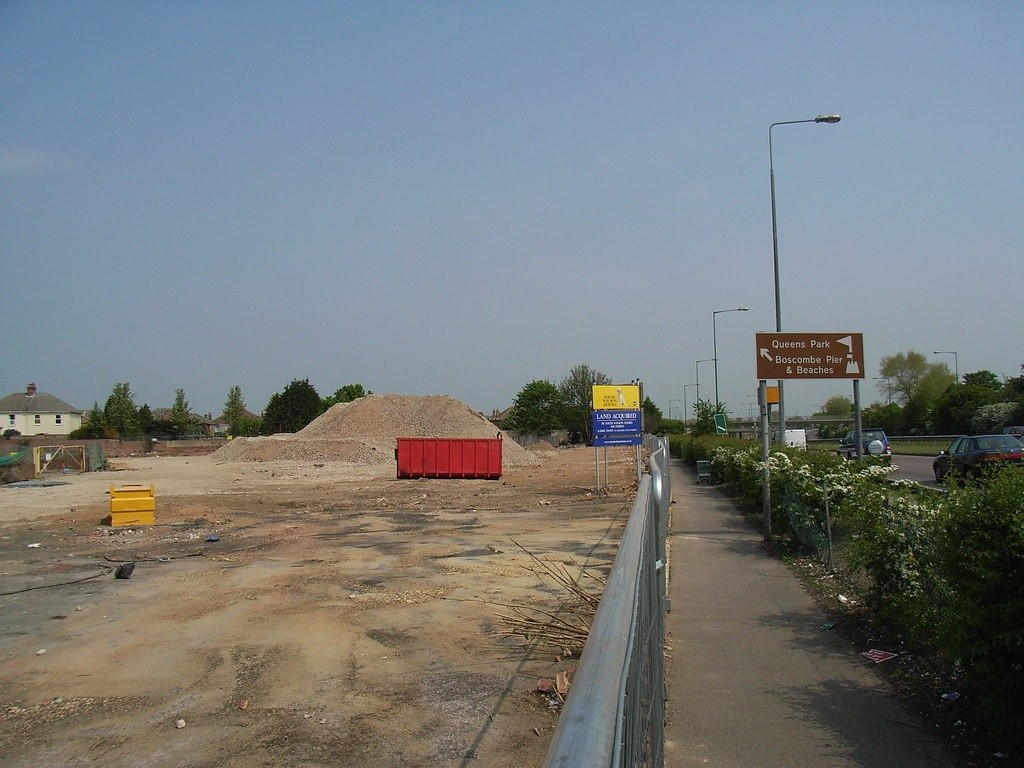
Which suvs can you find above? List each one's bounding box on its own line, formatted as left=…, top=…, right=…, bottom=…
left=837, top=428, right=891, bottom=466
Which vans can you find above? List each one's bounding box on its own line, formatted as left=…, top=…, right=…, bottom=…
left=772, top=428, right=807, bottom=451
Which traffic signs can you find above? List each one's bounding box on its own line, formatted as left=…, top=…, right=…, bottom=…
left=755, top=332, right=865, bottom=380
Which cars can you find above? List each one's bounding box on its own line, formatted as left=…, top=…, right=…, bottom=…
left=933, top=434, right=1024, bottom=486
left=1002, top=425, right=1024, bottom=438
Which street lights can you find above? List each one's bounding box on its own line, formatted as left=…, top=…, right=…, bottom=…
left=712, top=306, right=748, bottom=414
left=768, top=114, right=842, bottom=445
left=872, top=377, right=891, bottom=413
left=696, top=357, right=719, bottom=420
left=683, top=383, right=700, bottom=436
left=934, top=351, right=958, bottom=384
left=839, top=394, right=853, bottom=403
left=669, top=399, right=681, bottom=420
